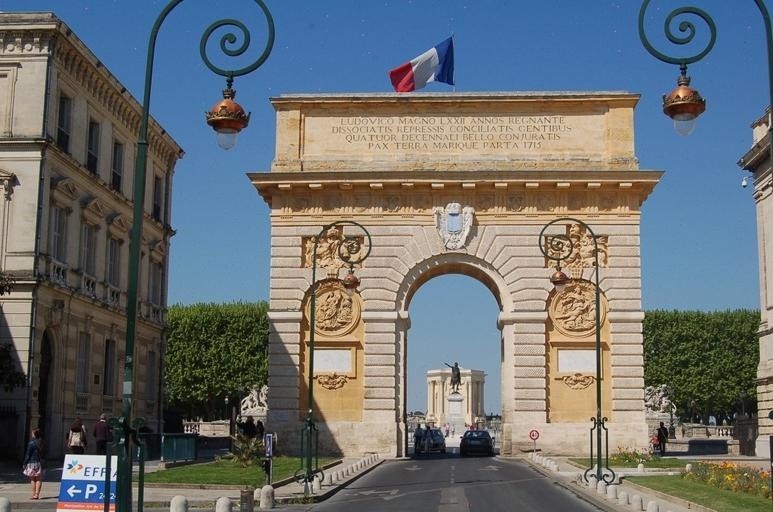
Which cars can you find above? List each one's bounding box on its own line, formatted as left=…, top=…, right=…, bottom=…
left=415, top=429, right=446, bottom=454
left=459, top=430, right=495, bottom=456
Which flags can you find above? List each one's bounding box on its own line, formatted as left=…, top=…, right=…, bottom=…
left=388, top=34, right=454, bottom=92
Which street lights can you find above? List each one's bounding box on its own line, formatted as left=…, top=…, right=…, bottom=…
left=637, top=0, right=773, bottom=137
left=105, top=0, right=275, bottom=512
left=295, top=219, right=371, bottom=485
left=538, top=218, right=616, bottom=484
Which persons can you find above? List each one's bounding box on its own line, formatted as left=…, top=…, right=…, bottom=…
left=93, top=412, right=111, bottom=454
left=651, top=421, right=666, bottom=457
left=68, top=414, right=88, bottom=454
left=443, top=361, right=462, bottom=394
left=22, top=428, right=44, bottom=500
left=413, top=414, right=500, bottom=455
left=233, top=413, right=264, bottom=440
